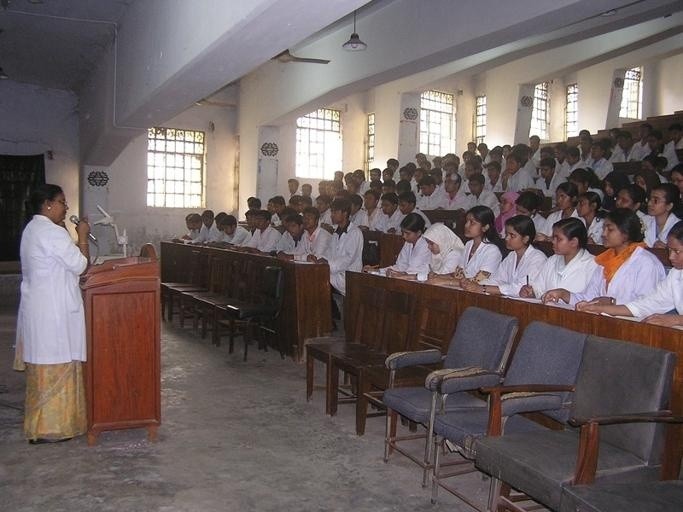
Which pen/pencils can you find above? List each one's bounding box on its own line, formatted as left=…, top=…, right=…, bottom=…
left=370, top=264, right=380, bottom=269
left=527, top=275, right=528, bottom=287
left=428, top=263, right=433, bottom=272
left=586, top=301, right=600, bottom=306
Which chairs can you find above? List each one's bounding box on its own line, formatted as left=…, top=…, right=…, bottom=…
left=562, top=413, right=683, bottom=512
left=156, top=109, right=683, bottom=488
left=480, top=333, right=676, bottom=512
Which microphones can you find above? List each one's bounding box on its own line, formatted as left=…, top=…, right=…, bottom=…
left=69, top=215, right=98, bottom=243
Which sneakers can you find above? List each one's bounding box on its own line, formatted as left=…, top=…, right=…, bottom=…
left=29, top=437, right=74, bottom=444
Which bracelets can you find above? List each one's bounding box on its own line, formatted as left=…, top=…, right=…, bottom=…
left=78, top=243, right=89, bottom=245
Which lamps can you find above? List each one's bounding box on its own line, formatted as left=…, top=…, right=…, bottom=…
left=339, top=9, right=367, bottom=53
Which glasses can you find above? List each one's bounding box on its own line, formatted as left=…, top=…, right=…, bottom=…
left=44, top=198, right=67, bottom=206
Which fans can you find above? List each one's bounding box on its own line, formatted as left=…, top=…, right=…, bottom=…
left=268, top=49, right=331, bottom=66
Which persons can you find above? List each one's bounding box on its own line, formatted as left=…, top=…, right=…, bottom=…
left=14, top=184, right=90, bottom=442
